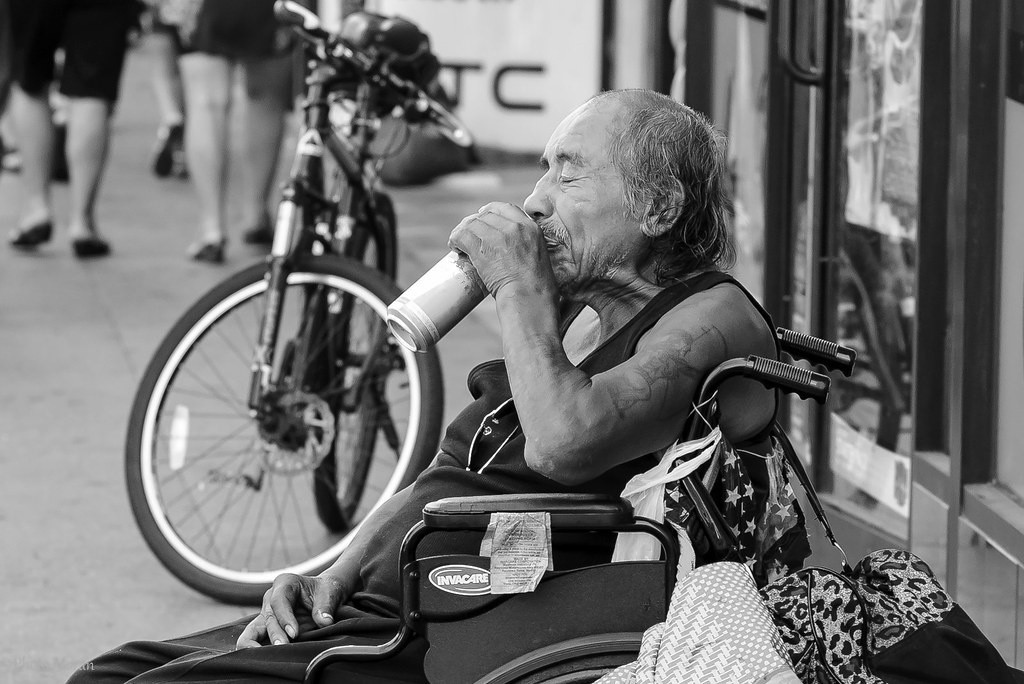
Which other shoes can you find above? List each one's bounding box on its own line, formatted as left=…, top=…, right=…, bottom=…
left=7, top=207, right=52, bottom=245
left=150, top=114, right=185, bottom=176
left=69, top=225, right=109, bottom=258
left=188, top=232, right=228, bottom=262
left=241, top=206, right=273, bottom=243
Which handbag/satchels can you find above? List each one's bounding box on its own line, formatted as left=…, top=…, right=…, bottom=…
left=757, top=549, right=1024, bottom=683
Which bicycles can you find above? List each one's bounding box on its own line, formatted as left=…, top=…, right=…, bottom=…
left=839, top=218, right=912, bottom=503
left=123, top=0, right=478, bottom=604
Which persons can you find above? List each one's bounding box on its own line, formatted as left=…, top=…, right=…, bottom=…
left=0, top=0, right=306, bottom=261
left=64, top=87, right=781, bottom=684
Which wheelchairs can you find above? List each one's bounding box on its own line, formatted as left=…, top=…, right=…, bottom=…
left=301, top=329, right=864, bottom=683
